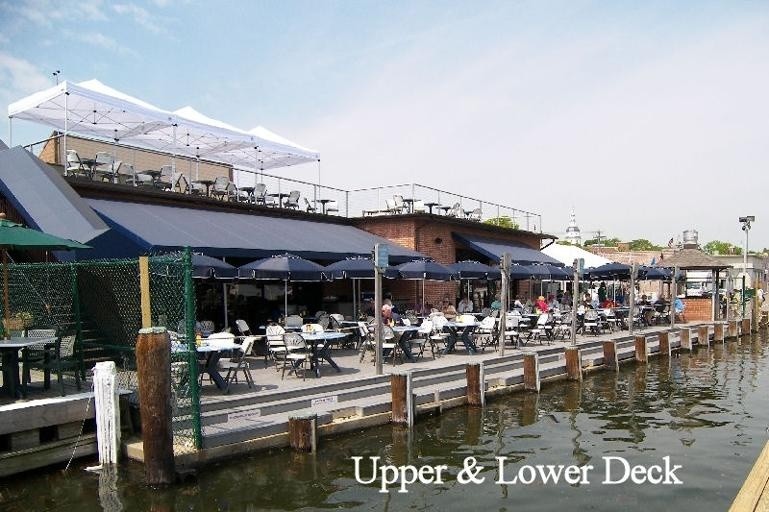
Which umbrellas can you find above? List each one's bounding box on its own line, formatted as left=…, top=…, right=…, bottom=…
left=0, top=211, right=93, bottom=373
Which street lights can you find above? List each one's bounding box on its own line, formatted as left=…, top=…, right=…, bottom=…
left=570, top=257, right=584, bottom=346
left=499, top=252, right=511, bottom=357
left=669, top=265, right=681, bottom=328
left=738, top=214, right=756, bottom=277
left=629, top=262, right=643, bottom=335
left=371, top=240, right=392, bottom=378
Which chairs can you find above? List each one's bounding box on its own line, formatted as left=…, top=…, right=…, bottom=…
left=360, top=194, right=484, bottom=223
left=62, top=150, right=340, bottom=217
left=0, top=300, right=675, bottom=409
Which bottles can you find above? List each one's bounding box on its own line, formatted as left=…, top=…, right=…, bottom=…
left=306, top=324, right=311, bottom=332
left=196, top=332, right=202, bottom=347
left=3, top=331, right=11, bottom=341
